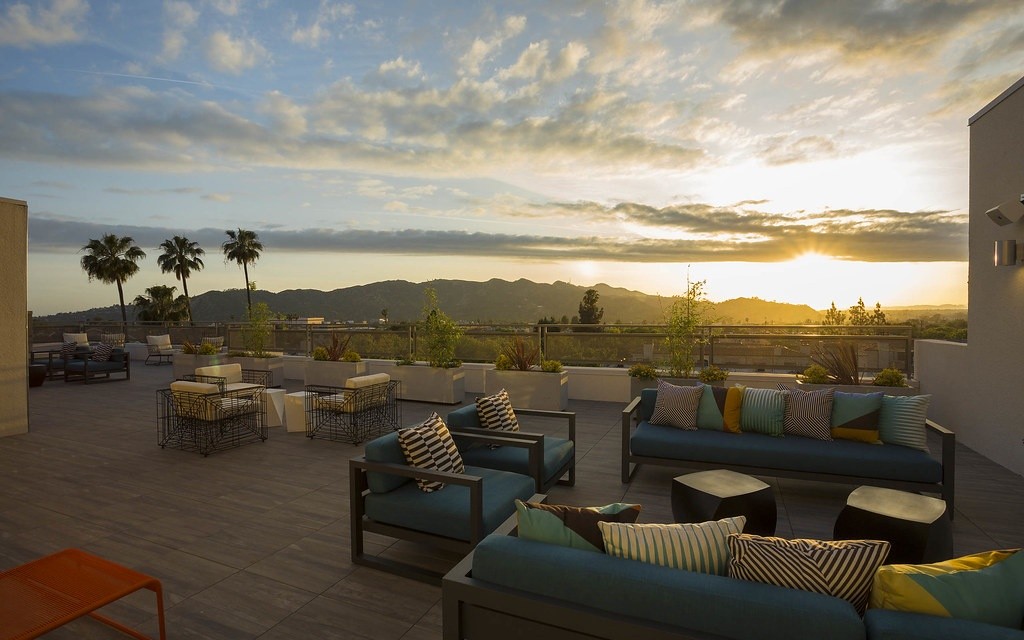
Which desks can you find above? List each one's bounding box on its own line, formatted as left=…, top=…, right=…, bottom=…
left=0, top=546, right=165, bottom=640
left=284, top=390, right=319, bottom=433
left=253, top=388, right=286, bottom=427
left=671, top=469, right=778, bottom=536
left=833, top=485, right=954, bottom=566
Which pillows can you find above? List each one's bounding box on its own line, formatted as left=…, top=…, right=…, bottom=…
left=475, top=387, right=519, bottom=450
left=514, top=499, right=1024, bottom=629
left=59, top=341, right=77, bottom=359
left=647, top=379, right=932, bottom=454
left=396, top=410, right=466, bottom=494
left=92, top=342, right=115, bottom=362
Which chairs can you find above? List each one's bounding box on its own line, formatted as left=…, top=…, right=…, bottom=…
left=29, top=333, right=130, bottom=387
left=145, top=334, right=172, bottom=365
left=348, top=422, right=539, bottom=586
left=304, top=372, right=402, bottom=446
left=157, top=363, right=273, bottom=457
left=445, top=402, right=576, bottom=495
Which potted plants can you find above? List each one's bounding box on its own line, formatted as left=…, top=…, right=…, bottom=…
left=627, top=280, right=730, bottom=418
left=795, top=341, right=916, bottom=397
left=224, top=302, right=284, bottom=387
left=173, top=335, right=228, bottom=375
left=484, top=332, right=569, bottom=412
left=303, top=326, right=369, bottom=392
left=388, top=287, right=465, bottom=404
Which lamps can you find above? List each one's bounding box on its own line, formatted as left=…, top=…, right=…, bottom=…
left=995, top=240, right=1024, bottom=266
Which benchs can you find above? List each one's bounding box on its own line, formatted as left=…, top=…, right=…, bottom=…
left=441, top=493, right=1024, bottom=640
left=622, top=395, right=956, bottom=520
